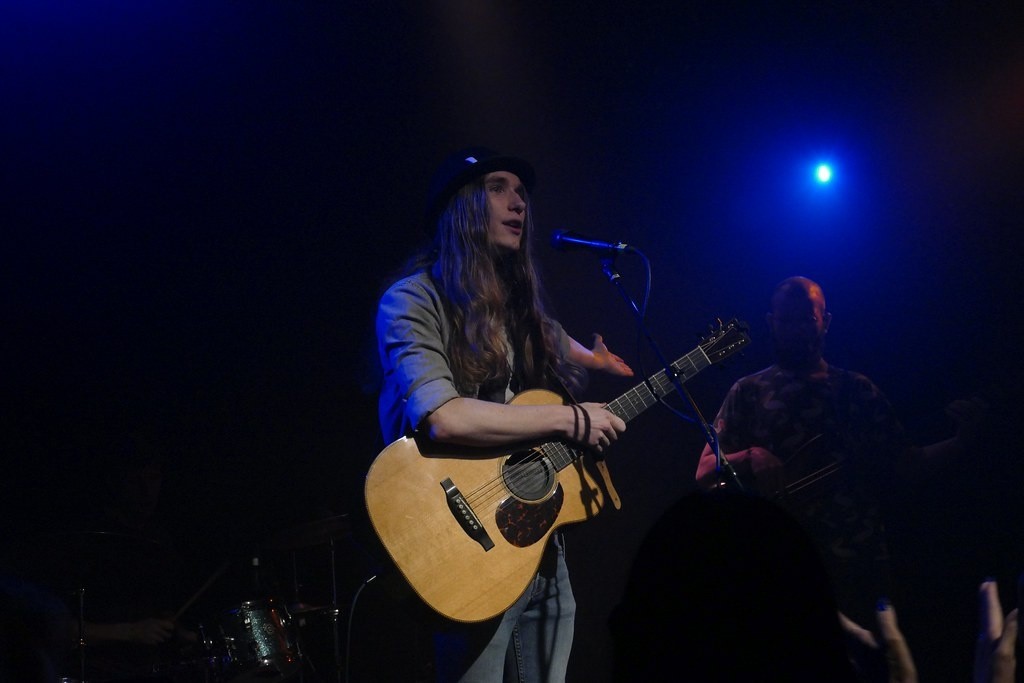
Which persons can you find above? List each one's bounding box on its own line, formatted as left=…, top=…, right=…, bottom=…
left=837, top=580, right=1018, bottom=682
left=58, top=612, right=201, bottom=648
left=374, top=151, right=634, bottom=682
left=695, top=276, right=987, bottom=618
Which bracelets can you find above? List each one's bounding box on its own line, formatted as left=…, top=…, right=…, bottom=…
left=578, top=404, right=591, bottom=444
left=568, top=404, right=578, bottom=440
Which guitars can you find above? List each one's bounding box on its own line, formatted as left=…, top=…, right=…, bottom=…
left=363, top=314, right=757, bottom=625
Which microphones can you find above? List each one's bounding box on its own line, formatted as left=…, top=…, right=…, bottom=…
left=550, top=228, right=636, bottom=259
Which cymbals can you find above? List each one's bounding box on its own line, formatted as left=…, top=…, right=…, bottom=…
left=287, top=601, right=329, bottom=616
left=280, top=508, right=348, bottom=535
left=52, top=520, right=161, bottom=551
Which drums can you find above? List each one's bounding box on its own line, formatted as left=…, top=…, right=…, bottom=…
left=193, top=593, right=306, bottom=683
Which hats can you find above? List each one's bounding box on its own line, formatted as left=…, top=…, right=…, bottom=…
left=423, top=150, right=533, bottom=196
